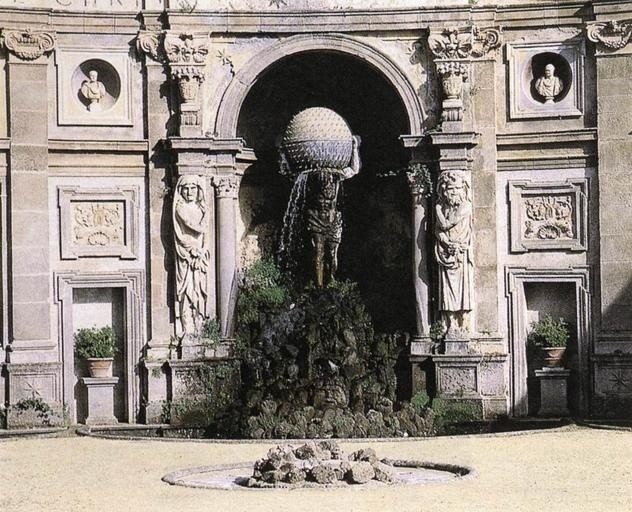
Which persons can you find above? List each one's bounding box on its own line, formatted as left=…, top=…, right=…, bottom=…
left=275, top=132, right=364, bottom=291
left=433, top=170, right=476, bottom=331
left=79, top=70, right=108, bottom=102
left=535, top=64, right=564, bottom=97
left=171, top=173, right=213, bottom=337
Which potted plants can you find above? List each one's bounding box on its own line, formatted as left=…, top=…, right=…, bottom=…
left=73, top=326, right=121, bottom=378
left=528, top=311, right=571, bottom=368
